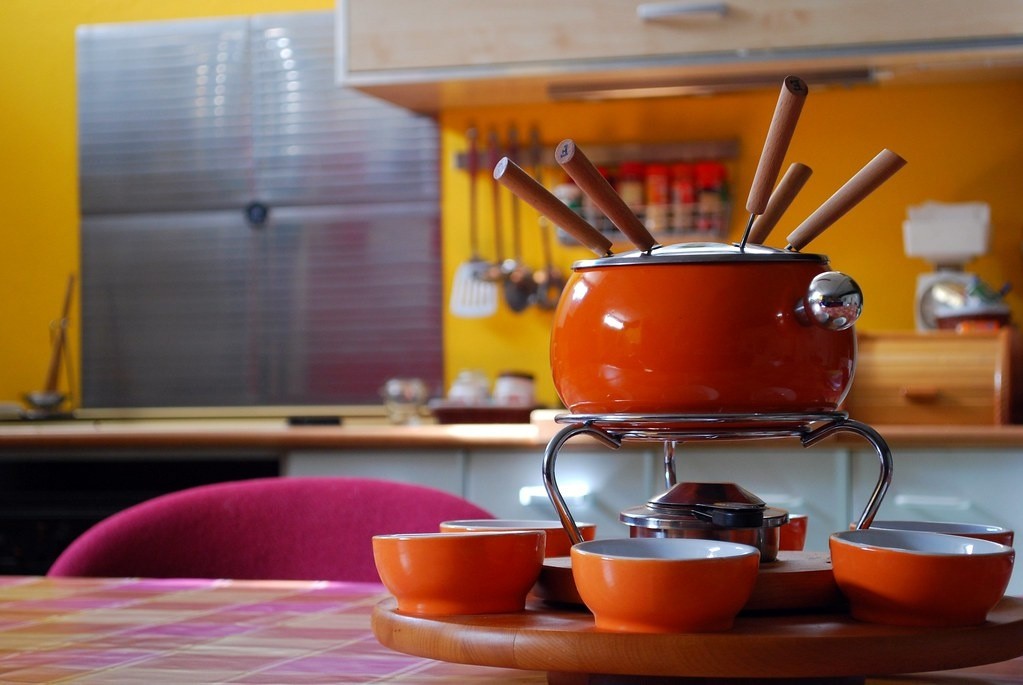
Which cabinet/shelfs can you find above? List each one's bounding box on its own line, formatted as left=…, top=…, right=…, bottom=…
left=292, top=446, right=1023, bottom=596
left=326, top=0, right=1023, bottom=111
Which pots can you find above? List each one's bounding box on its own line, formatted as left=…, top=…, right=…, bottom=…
left=618, top=484, right=788, bottom=563
left=547, top=239, right=858, bottom=431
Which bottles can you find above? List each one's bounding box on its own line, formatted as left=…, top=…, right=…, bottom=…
left=553, top=159, right=727, bottom=236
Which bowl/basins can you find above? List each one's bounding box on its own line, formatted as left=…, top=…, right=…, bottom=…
left=849, top=520, right=1015, bottom=549
left=570, top=537, right=760, bottom=631
left=828, top=529, right=1015, bottom=627
left=372, top=530, right=546, bottom=616
left=779, top=513, right=808, bottom=552
left=439, top=519, right=597, bottom=560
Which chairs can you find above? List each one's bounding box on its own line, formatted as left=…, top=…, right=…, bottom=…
left=49, top=476, right=503, bottom=583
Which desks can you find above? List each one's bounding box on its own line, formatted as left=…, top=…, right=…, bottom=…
left=1, top=576, right=1023, bottom=685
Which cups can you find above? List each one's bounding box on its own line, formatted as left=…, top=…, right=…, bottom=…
left=383, top=377, right=427, bottom=422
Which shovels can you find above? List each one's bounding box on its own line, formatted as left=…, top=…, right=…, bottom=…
left=448, top=123, right=497, bottom=316
